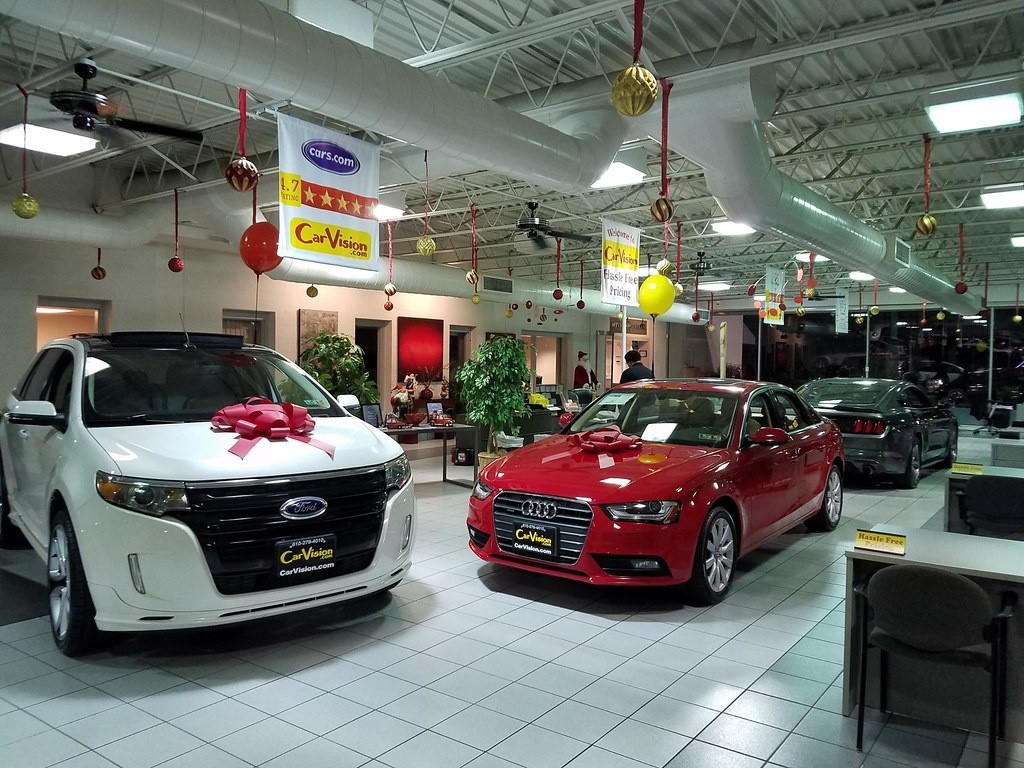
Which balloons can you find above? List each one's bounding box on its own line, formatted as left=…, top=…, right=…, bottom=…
left=240, top=222, right=283, bottom=274
left=638, top=275, right=675, bottom=317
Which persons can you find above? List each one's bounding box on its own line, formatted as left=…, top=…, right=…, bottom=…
left=525, top=369, right=542, bottom=387
left=574, top=350, right=602, bottom=401
left=902, top=358, right=949, bottom=406
left=620, top=350, right=656, bottom=408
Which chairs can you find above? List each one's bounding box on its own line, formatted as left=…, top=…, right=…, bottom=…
left=968, top=370, right=1017, bottom=436
left=713, top=398, right=761, bottom=436
left=688, top=399, right=718, bottom=428
left=956, top=475, right=1024, bottom=541
left=851, top=563, right=1013, bottom=768
left=1012, top=421, right=1024, bottom=427
left=759, top=403, right=791, bottom=432
left=998, top=432, right=1020, bottom=439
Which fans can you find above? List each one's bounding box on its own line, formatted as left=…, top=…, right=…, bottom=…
left=474, top=202, right=593, bottom=250
left=803, top=291, right=845, bottom=301
left=0, top=56, right=204, bottom=144
left=671, top=252, right=743, bottom=283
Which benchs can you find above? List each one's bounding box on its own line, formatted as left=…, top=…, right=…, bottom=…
left=943, top=466, right=1024, bottom=541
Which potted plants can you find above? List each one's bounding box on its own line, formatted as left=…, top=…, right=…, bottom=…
left=454, top=334, right=538, bottom=471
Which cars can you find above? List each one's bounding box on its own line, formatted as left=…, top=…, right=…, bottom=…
left=807, top=337, right=906, bottom=370
left=467, top=377, right=847, bottom=606
left=796, top=378, right=957, bottom=490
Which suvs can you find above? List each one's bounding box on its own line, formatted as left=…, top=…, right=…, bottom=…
left=897, top=360, right=965, bottom=393
left=0, top=327, right=419, bottom=657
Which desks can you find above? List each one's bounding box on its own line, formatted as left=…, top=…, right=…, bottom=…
left=375, top=423, right=478, bottom=489
left=991, top=438, right=1024, bottom=468
left=1001, top=426, right=1024, bottom=438
left=842, top=523, right=1024, bottom=744
